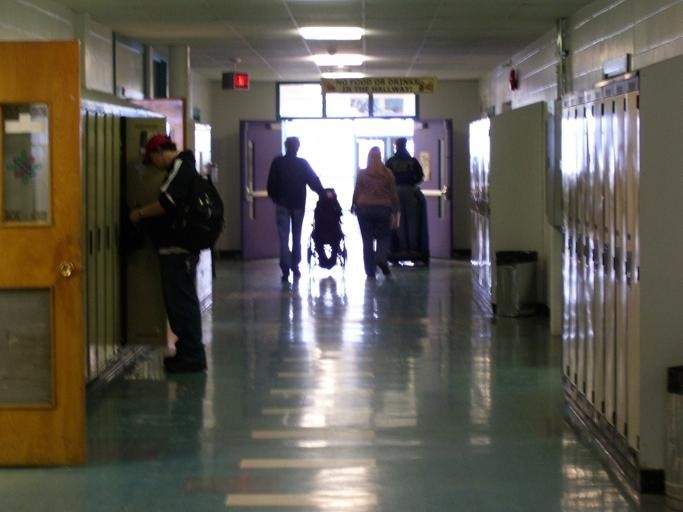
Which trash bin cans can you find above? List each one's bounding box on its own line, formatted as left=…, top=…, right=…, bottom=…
left=497, top=251, right=538, bottom=317
left=669, top=366, right=682, bottom=504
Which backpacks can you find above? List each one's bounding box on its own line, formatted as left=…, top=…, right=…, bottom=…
left=170, top=153, right=224, bottom=251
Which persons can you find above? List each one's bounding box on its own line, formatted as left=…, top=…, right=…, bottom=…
left=348, top=137, right=424, bottom=278
left=267, top=136, right=343, bottom=280
left=127, top=134, right=208, bottom=375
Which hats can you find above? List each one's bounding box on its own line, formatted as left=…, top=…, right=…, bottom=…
left=141, top=133, right=174, bottom=167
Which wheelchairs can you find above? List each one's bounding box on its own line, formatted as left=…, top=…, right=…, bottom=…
left=306, top=222, right=348, bottom=268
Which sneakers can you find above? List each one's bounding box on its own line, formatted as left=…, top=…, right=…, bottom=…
left=282, top=267, right=301, bottom=280
left=162, top=355, right=207, bottom=375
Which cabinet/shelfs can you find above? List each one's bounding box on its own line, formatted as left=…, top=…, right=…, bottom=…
left=79, top=114, right=119, bottom=399
left=558, top=77, right=639, bottom=454
left=466, top=100, right=541, bottom=321
